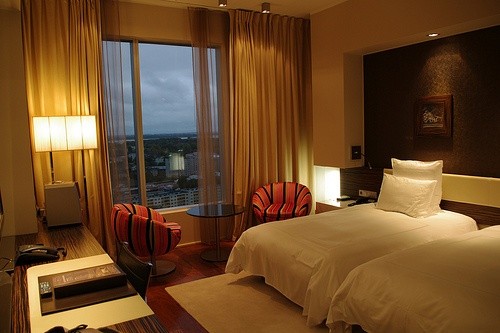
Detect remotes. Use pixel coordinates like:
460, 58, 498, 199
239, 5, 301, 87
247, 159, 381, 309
40, 281, 52, 298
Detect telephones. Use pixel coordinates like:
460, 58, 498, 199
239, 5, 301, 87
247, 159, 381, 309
15, 245, 60, 265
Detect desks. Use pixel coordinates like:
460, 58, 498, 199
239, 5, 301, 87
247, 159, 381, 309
186, 204, 246, 263
0, 223, 166, 333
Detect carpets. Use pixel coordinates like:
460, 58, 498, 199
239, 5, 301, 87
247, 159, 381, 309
164, 273, 323, 333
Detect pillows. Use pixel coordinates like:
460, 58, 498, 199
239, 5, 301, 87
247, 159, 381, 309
375, 158, 443, 217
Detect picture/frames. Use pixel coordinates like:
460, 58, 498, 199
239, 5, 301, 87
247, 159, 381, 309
413, 94, 453, 139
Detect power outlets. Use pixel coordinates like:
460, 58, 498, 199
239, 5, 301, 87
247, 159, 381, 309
352, 146, 361, 160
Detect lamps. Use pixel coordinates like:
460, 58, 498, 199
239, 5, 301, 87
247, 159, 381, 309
32, 114, 98, 184
219, 0, 227, 8
262, 2, 270, 14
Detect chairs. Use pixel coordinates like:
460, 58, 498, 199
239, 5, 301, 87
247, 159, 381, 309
251, 182, 312, 225
111, 204, 182, 276
225, 166, 500, 333
